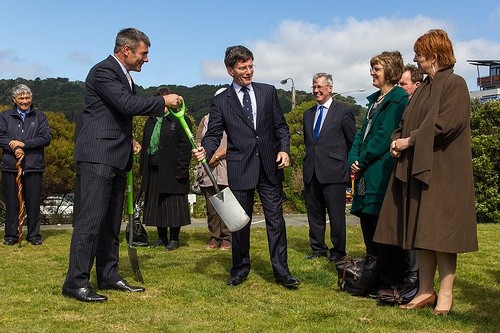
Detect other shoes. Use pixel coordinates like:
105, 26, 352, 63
218, 243, 231, 250
3, 239, 19, 245
206, 244, 218, 251
166, 239, 179, 249
153, 237, 168, 247
399, 291, 438, 310
326, 255, 346, 263
305, 253, 328, 259
30, 238, 42, 245
433, 302, 453, 315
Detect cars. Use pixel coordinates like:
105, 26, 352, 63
40, 196, 74, 219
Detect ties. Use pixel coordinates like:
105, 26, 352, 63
241, 87, 254, 128
314, 106, 325, 140
126, 73, 132, 90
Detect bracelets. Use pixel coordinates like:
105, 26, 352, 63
215, 152, 220, 161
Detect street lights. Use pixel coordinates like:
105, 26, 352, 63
280, 78, 296, 110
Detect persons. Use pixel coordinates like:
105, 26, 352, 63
348, 50, 409, 271
0, 84, 52, 245
396, 62, 423, 285
139, 87, 192, 250
62, 27, 182, 302
303, 73, 356, 261
190, 45, 301, 288
190, 87, 232, 251
373, 30, 478, 316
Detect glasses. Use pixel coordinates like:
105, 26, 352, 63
370, 67, 384, 72
310, 85, 330, 89
415, 54, 423, 59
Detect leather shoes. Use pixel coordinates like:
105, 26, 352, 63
227, 275, 247, 286
104, 279, 146, 292
62, 286, 108, 303
274, 275, 301, 287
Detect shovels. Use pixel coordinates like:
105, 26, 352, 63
167, 95, 251, 233
126, 147, 145, 284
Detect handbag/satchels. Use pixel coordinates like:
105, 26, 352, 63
335, 255, 378, 291
376, 285, 418, 305
191, 182, 205, 195
125, 191, 148, 246
